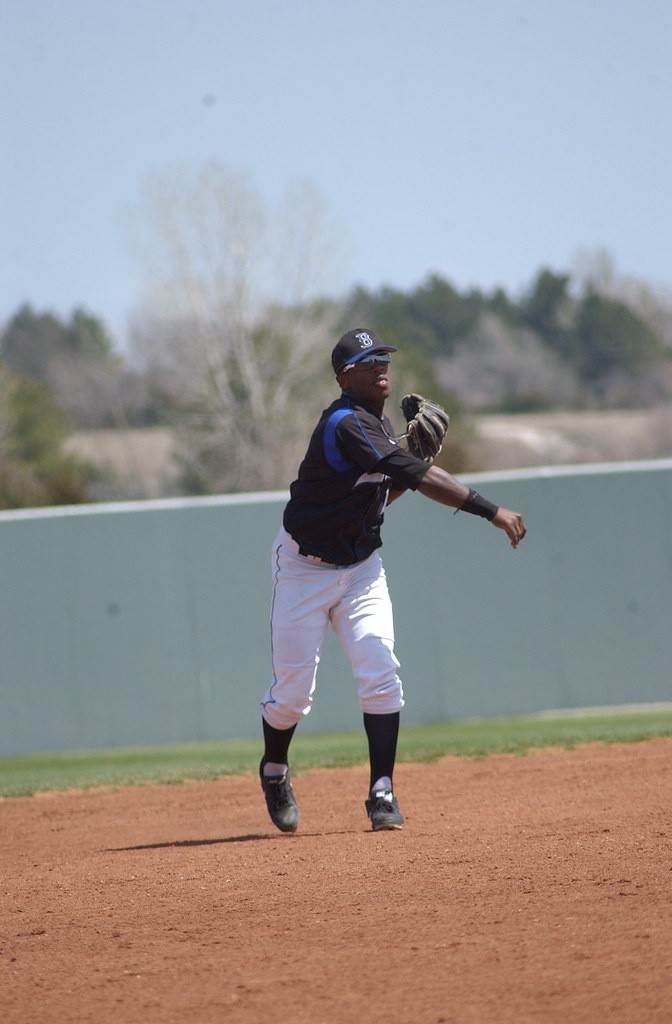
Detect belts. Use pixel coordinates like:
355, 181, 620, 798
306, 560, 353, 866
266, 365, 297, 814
298, 546, 335, 564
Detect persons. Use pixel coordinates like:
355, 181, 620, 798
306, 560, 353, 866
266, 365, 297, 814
259, 328, 527, 832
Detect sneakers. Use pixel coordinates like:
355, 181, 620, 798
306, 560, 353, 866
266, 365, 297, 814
261, 769, 300, 833
366, 790, 404, 830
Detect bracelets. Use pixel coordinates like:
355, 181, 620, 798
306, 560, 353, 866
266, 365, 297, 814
460, 487, 498, 521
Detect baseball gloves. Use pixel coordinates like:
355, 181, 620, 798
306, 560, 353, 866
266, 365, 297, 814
398, 392, 451, 464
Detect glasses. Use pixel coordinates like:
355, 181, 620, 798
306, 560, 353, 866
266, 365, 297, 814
341, 351, 391, 372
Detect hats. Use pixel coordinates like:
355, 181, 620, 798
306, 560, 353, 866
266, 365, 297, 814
332, 329, 398, 375
